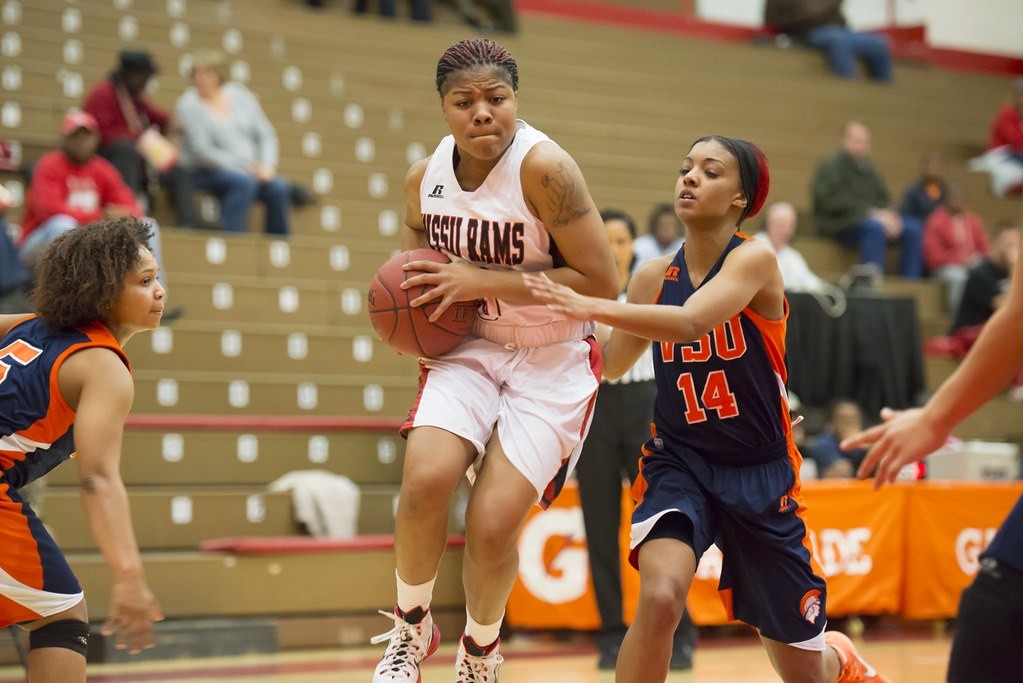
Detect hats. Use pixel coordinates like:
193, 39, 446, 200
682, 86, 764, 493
62, 110, 98, 136
116, 48, 156, 74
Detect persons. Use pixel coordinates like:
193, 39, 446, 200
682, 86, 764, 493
572, 206, 699, 671
754, 199, 826, 295
80, 51, 173, 211
838, 215, 1023, 683
0, 215, 165, 683
807, 117, 927, 286
370, 41, 624, 681
922, 199, 1023, 400
628, 204, 686, 262
987, 94, 1023, 195
526, 137, 884, 683
16, 109, 165, 288
784, 400, 961, 481
168, 50, 293, 234
763, 1, 895, 85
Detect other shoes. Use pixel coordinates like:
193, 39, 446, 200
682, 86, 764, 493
598, 641, 619, 669
670, 643, 694, 671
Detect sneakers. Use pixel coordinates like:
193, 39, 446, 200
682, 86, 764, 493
827, 632, 886, 683
371, 606, 441, 683
455, 631, 504, 683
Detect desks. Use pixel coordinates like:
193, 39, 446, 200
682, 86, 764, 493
498, 479, 1020, 646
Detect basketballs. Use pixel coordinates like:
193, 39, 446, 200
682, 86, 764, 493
367, 247, 478, 360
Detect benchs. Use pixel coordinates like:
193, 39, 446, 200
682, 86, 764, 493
0, 0, 1023, 623
